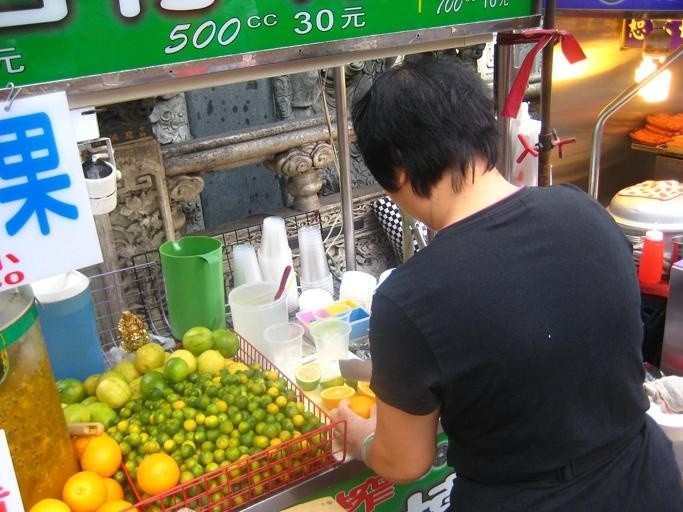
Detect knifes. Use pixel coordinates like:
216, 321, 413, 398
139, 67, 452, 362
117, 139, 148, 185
338, 356, 373, 382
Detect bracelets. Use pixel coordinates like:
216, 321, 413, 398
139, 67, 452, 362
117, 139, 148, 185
360, 430, 374, 469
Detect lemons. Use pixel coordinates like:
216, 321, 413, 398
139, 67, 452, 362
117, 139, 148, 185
28, 430, 180, 512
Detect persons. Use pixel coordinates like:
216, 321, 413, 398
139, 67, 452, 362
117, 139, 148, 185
329, 51, 681, 511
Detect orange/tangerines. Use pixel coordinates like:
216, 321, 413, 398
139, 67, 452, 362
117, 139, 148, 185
56, 326, 329, 512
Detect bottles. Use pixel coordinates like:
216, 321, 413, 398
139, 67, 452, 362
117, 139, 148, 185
81, 138, 112, 180
639, 231, 664, 284
29, 268, 107, 380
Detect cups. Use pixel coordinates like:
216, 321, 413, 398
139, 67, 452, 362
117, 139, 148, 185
297, 226, 335, 309
338, 265, 396, 312
231, 215, 299, 313
226, 280, 306, 377
159, 235, 227, 343
308, 301, 353, 377
